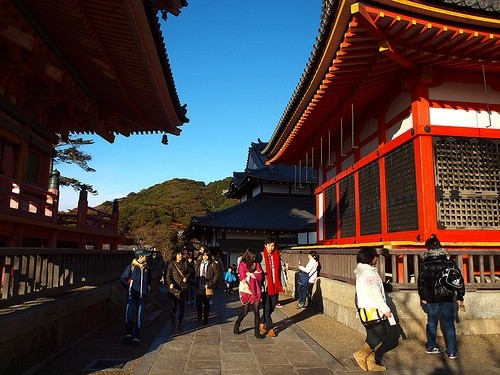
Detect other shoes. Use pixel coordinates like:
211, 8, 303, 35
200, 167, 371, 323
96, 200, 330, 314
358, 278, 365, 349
259, 323, 268, 333
203, 318, 209, 325
171, 317, 175, 325
198, 317, 202, 322
178, 323, 183, 329
269, 328, 276, 337
275, 303, 283, 308
132, 338, 141, 347
295, 302, 305, 309
122, 334, 132, 344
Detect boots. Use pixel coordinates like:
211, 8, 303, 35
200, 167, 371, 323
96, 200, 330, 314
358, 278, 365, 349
353, 342, 373, 371
254, 328, 263, 339
366, 352, 387, 372
234, 322, 242, 334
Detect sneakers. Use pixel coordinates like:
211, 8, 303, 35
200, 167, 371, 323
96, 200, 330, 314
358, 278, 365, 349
448, 352, 459, 359
426, 344, 444, 354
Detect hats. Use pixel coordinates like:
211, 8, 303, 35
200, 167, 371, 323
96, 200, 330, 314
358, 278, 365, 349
135, 249, 151, 256
425, 238, 441, 249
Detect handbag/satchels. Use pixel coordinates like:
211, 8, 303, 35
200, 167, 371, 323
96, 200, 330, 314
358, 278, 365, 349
239, 281, 252, 295
439, 257, 465, 298
206, 288, 215, 299
297, 272, 309, 287
356, 308, 383, 329
109, 267, 132, 307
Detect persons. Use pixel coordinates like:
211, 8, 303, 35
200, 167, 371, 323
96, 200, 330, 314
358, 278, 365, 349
353, 247, 391, 372
195, 246, 223, 325
275, 303, 284, 308
417, 237, 466, 359
150, 251, 166, 293
120, 249, 151, 343
234, 247, 265, 339
297, 251, 319, 308
260, 236, 287, 337
166, 248, 191, 329
224, 267, 237, 295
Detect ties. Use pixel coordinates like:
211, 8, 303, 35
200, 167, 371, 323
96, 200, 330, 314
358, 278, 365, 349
201, 263, 207, 284
